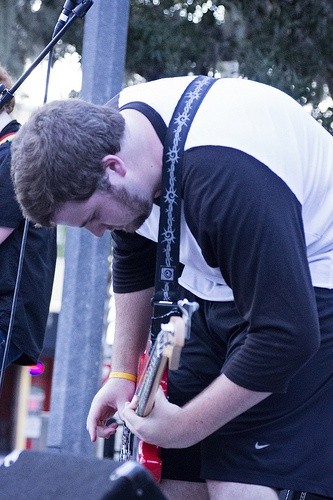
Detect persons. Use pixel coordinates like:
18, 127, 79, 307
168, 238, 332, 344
0, 66, 58, 456
11, 75, 333, 500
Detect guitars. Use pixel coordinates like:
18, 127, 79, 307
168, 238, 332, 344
112, 297, 199, 483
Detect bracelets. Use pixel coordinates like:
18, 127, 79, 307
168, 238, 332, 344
109, 372, 137, 384
